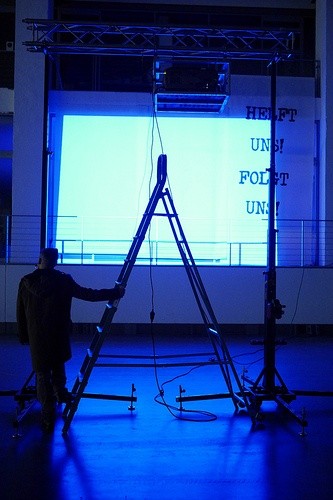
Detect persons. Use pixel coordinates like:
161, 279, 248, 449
16, 247, 126, 426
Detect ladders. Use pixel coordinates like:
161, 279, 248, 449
61, 153, 256, 436
